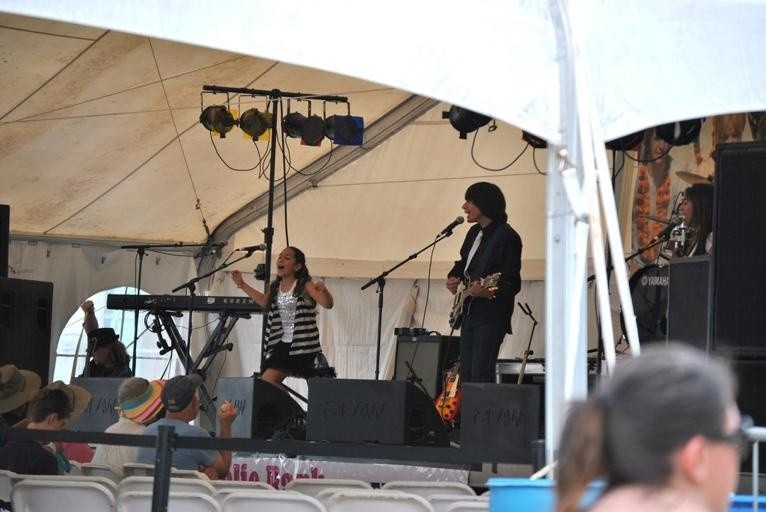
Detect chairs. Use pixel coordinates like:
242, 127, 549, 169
1, 460, 491, 511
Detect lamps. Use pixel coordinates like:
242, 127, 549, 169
199, 84, 364, 146
655, 118, 702, 147
607, 130, 645, 151
523, 130, 545, 147
443, 103, 492, 139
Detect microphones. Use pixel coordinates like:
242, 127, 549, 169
439, 217, 464, 237
237, 243, 266, 251
219, 239, 227, 248
650, 219, 678, 244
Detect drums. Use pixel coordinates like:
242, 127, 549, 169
619, 264, 669, 348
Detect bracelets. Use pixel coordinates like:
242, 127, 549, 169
237, 281, 246, 288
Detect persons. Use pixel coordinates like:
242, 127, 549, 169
556, 340, 751, 512
697, 113, 753, 178
78, 299, 134, 378
0, 365, 240, 478
446, 182, 523, 384
633, 128, 686, 263
231, 247, 334, 395
679, 183, 716, 258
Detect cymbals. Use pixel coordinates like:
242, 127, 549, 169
675, 172, 714, 185
641, 214, 673, 224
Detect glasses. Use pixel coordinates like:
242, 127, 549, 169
687, 416, 750, 461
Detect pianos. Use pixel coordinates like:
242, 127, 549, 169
107, 294, 263, 315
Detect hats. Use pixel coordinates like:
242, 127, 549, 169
465, 182, 508, 223
162, 374, 203, 412
26, 380, 91, 428
0, 364, 41, 415
85, 327, 119, 351
113, 378, 168, 425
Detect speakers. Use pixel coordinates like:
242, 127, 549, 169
0, 205, 10, 278
215, 377, 306, 442
729, 359, 766, 493
461, 382, 543, 473
392, 334, 459, 403
66, 377, 126, 433
712, 141, 765, 359
306, 376, 450, 447
1, 278, 53, 389
665, 255, 713, 364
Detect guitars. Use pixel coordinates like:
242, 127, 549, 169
449, 272, 501, 329
434, 356, 462, 421
